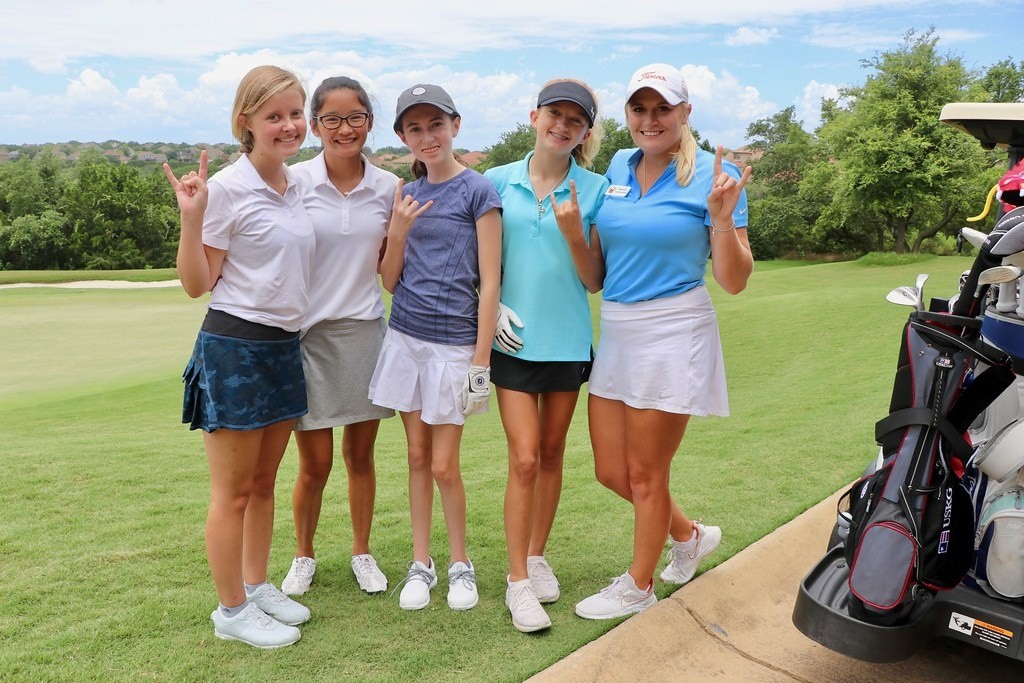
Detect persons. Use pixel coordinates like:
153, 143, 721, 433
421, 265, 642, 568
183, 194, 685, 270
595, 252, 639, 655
380, 84, 503, 611
482, 78, 610, 634
163, 65, 316, 650
573, 63, 754, 621
281, 77, 401, 596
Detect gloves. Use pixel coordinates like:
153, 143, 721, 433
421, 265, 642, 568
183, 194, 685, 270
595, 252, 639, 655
494, 301, 524, 353
462, 366, 491, 414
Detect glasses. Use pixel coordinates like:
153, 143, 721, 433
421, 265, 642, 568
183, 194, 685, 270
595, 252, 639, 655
313, 113, 370, 130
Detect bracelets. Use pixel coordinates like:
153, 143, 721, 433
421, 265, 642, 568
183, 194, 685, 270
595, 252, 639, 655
710, 214, 734, 235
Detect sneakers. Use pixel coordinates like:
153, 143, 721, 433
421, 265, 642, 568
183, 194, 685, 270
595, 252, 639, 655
447, 557, 478, 611
399, 557, 437, 610
505, 574, 551, 632
525, 555, 561, 603
244, 582, 311, 626
211, 602, 302, 650
351, 554, 388, 592
575, 571, 659, 619
660, 523, 721, 583
281, 556, 316, 595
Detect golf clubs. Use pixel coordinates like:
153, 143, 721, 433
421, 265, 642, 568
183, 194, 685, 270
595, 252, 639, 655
961, 265, 1024, 337
885, 272, 934, 313
959, 227, 989, 250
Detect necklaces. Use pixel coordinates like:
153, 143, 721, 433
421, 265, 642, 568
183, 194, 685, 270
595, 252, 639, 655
325, 159, 362, 196
642, 154, 673, 192
529, 154, 570, 219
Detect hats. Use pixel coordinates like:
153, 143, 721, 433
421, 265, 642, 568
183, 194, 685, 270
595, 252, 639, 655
625, 63, 689, 106
395, 84, 457, 123
537, 82, 596, 128
